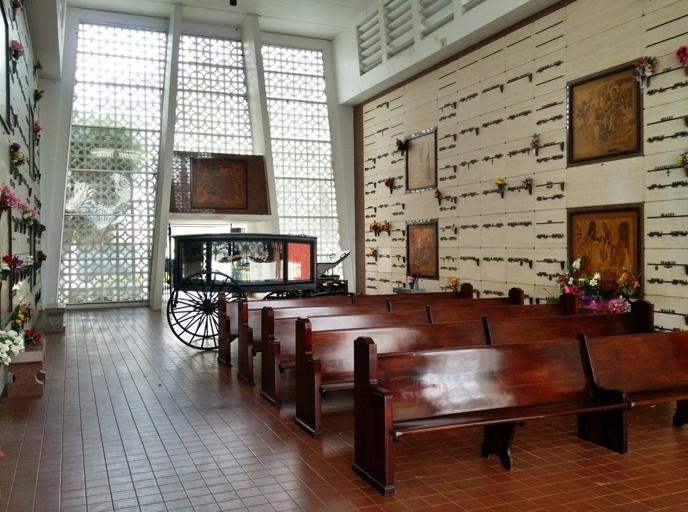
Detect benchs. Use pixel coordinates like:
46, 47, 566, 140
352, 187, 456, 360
218, 284, 687, 496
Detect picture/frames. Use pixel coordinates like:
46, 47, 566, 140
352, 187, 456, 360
189, 157, 248, 209
405, 124, 440, 197
561, 197, 651, 297
563, 57, 646, 172
404, 215, 442, 283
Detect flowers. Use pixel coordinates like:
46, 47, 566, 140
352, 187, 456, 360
444, 274, 462, 292
632, 43, 688, 178
492, 131, 545, 203
0, 0, 47, 366
551, 261, 651, 312
366, 132, 405, 262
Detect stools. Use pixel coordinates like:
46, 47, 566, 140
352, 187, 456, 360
43, 302, 67, 333
6, 350, 46, 397
